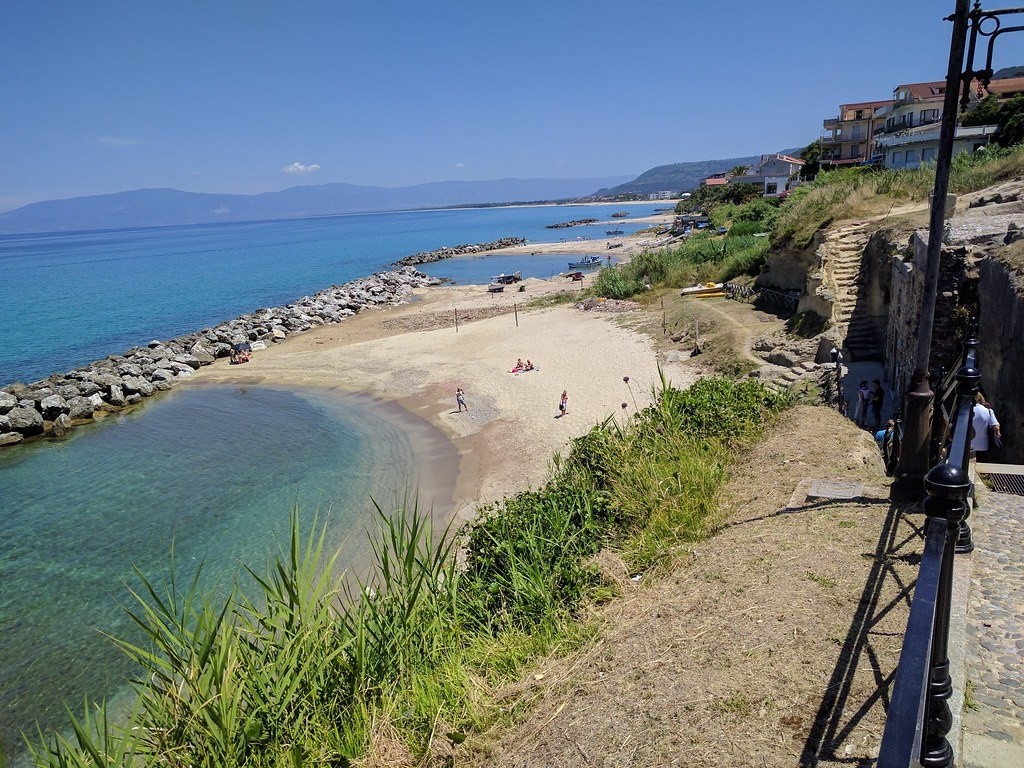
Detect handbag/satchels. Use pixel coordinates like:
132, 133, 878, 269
560, 404, 564, 410
987, 408, 1003, 450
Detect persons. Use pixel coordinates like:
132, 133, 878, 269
524, 359, 533, 370
520, 223, 684, 265
870, 379, 884, 430
561, 390, 568, 416
516, 358, 524, 370
456, 387, 468, 411
859, 421, 873, 434
969, 392, 1000, 463
854, 380, 871, 426
874, 419, 903, 462
939, 423, 956, 461
229, 348, 253, 364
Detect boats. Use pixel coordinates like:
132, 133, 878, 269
488, 282, 504, 293
491, 271, 522, 284
568, 255, 603, 268
604, 227, 624, 235
560, 269, 585, 281
